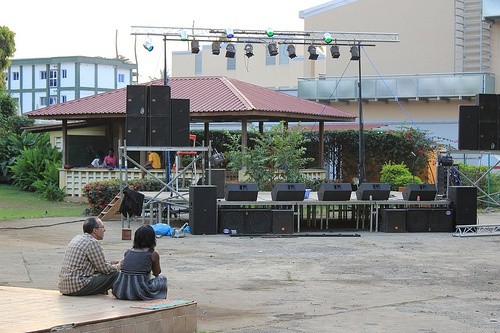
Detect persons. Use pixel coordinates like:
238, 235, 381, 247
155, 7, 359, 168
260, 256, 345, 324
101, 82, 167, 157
91, 148, 162, 180
58, 218, 119, 296
110, 224, 167, 299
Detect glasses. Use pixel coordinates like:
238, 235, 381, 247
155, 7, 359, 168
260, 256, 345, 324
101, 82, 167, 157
94, 225, 104, 228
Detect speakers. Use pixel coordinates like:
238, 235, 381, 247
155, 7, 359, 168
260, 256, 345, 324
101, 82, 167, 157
317, 183, 352, 201
147, 85, 171, 146
224, 184, 258, 201
379, 208, 406, 233
126, 85, 147, 146
171, 99, 190, 146
448, 186, 477, 232
476, 94, 500, 150
271, 183, 306, 201
189, 185, 218, 234
356, 183, 390, 200
458, 104, 479, 150
406, 209, 455, 232
218, 209, 294, 235
205, 169, 226, 198
402, 184, 437, 201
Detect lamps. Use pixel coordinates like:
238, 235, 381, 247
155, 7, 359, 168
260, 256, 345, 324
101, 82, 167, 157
330, 46, 340, 59
143, 42, 153, 51
225, 44, 236, 58
307, 46, 319, 60
266, 27, 274, 37
181, 31, 188, 40
212, 42, 221, 55
287, 45, 296, 59
323, 33, 332, 43
244, 44, 254, 58
226, 26, 234, 38
350, 46, 360, 60
191, 40, 200, 54
268, 43, 278, 57
